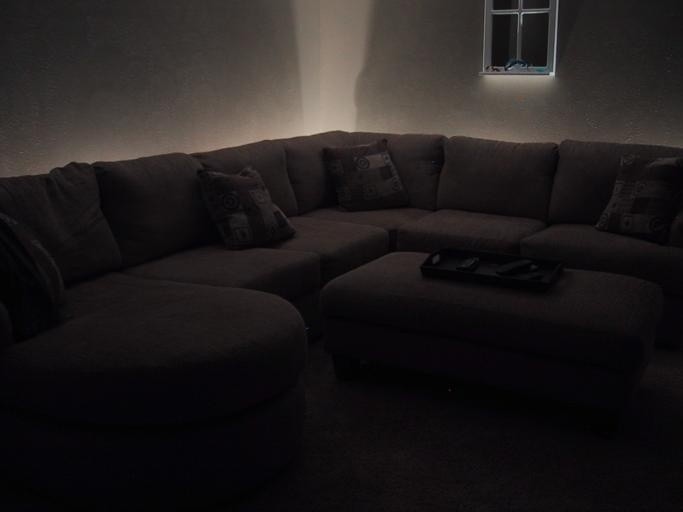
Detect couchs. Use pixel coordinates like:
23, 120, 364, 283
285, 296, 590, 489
3, 128, 683, 512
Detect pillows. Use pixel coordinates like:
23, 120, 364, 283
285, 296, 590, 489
321, 137, 410, 211
193, 165, 296, 249
597, 152, 682, 248
